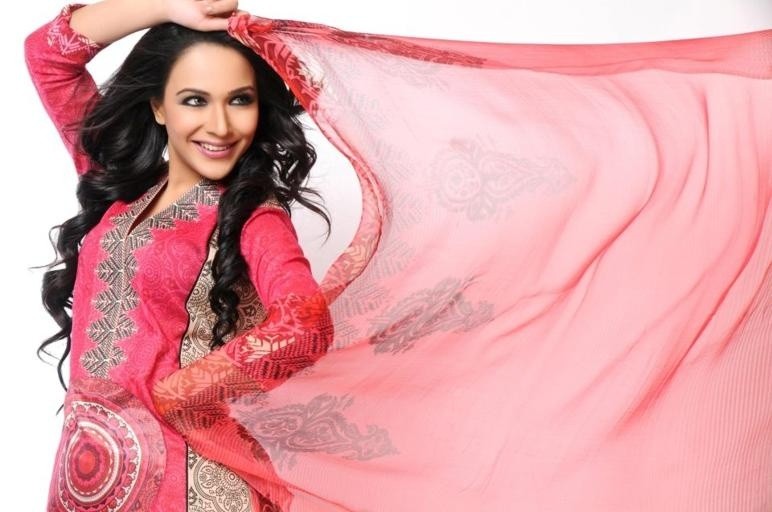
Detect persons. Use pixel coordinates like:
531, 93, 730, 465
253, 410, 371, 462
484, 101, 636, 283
24, 1, 335, 512
